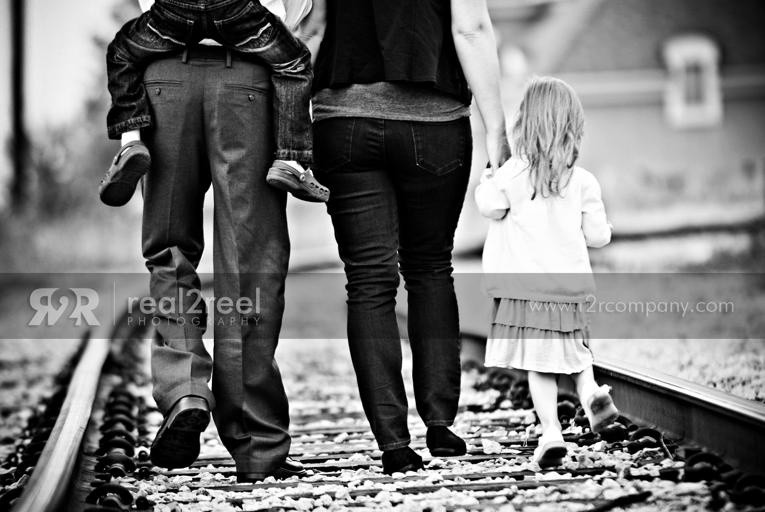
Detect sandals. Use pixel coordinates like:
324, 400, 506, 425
267, 159, 332, 203
584, 387, 619, 434
534, 435, 567, 467
98, 140, 153, 207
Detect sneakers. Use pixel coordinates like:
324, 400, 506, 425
151, 395, 209, 468
427, 426, 467, 457
237, 450, 306, 483
381, 447, 423, 474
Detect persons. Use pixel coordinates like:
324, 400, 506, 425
238, 17, 239, 21
142, 0, 317, 482
300, 0, 512, 477
474, 78, 618, 468
98, 0, 331, 207
